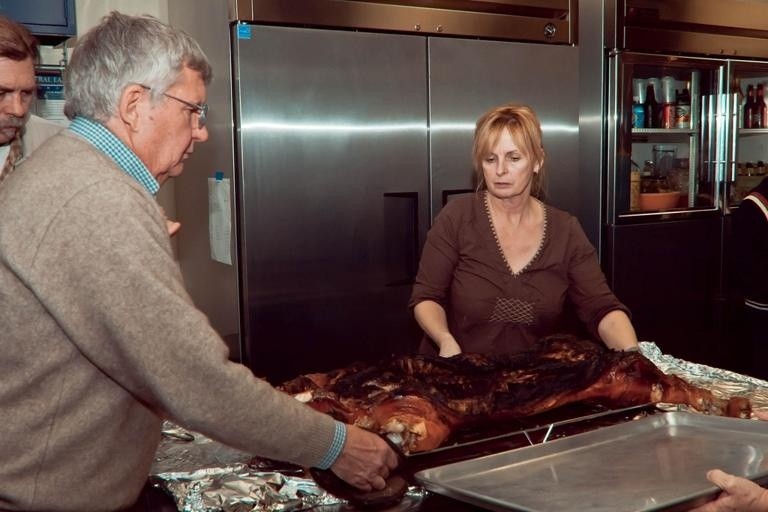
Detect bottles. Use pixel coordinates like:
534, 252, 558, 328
744, 83, 768, 129
632, 76, 692, 129
655, 143, 678, 176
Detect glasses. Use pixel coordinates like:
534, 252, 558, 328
123, 82, 209, 131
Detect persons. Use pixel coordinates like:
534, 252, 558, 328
0, 17, 402, 511
682, 468, 768, 512
1, 13, 65, 182
728, 176, 768, 383
409, 102, 646, 361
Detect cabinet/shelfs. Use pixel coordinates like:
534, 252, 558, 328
581, 1, 768, 305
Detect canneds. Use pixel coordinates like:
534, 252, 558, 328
639, 171, 654, 194
630, 76, 692, 130
644, 160, 656, 174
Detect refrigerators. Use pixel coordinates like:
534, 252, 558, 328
168, 0, 604, 388
